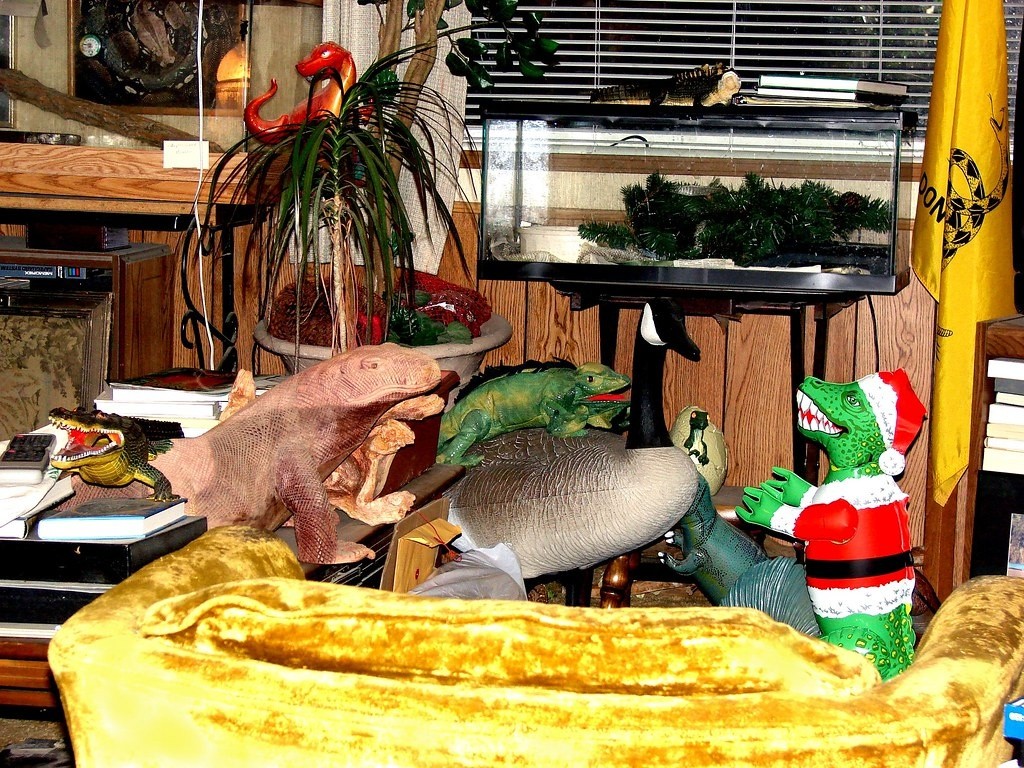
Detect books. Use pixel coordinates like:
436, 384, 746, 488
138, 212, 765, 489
982, 357, 1024, 475
94, 367, 289, 438
732, 74, 909, 108
0, 471, 208, 638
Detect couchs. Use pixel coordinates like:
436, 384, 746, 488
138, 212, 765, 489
48, 528, 1024, 768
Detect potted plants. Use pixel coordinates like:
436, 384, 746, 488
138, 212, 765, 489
169, 0, 564, 417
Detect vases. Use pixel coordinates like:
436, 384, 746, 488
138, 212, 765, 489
377, 370, 460, 498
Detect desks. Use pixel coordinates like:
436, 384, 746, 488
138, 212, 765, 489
551, 281, 870, 603
0, 191, 267, 373
0, 464, 466, 706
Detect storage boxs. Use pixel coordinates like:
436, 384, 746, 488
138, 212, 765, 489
477, 102, 919, 295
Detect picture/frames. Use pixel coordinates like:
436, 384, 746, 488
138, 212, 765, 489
66, 0, 252, 117
0, 15, 17, 129
0, 290, 114, 443
